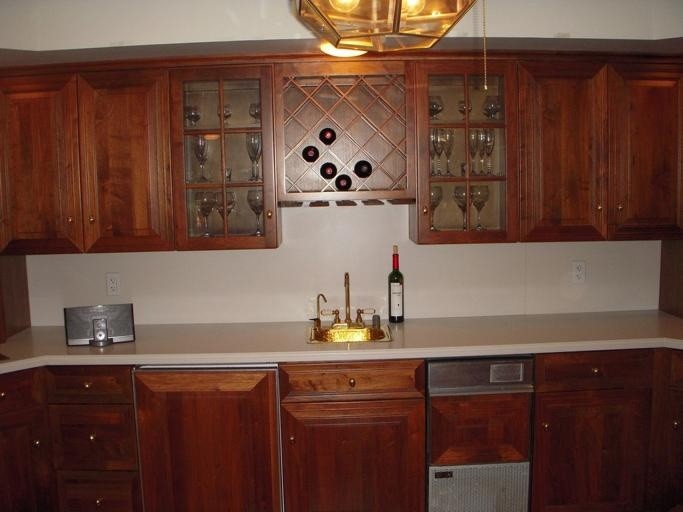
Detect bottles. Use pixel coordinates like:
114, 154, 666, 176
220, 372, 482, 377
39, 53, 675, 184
388, 246, 404, 323
303, 128, 372, 191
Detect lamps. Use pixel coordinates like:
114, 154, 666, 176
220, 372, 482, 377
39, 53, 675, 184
299, 1, 480, 53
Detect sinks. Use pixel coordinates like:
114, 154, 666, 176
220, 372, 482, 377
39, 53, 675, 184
305, 321, 393, 344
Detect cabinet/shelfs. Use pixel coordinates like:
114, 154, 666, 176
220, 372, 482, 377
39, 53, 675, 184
0, 60, 173, 252
656, 347, 683, 512
135, 366, 281, 512
170, 55, 281, 251
43, 363, 142, 511
406, 56, 516, 245
0, 368, 59, 512
516, 60, 682, 243
282, 359, 428, 512
533, 346, 655, 512
426, 359, 534, 512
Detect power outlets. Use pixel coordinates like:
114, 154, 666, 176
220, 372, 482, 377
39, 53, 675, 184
572, 260, 586, 282
106, 272, 121, 296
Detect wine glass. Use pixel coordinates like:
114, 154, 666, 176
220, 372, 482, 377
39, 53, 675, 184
458, 100, 472, 121
193, 135, 210, 183
429, 95, 444, 121
246, 133, 263, 181
470, 185, 489, 231
454, 186, 472, 231
482, 94, 501, 120
247, 190, 264, 237
429, 186, 443, 231
214, 192, 235, 220
428, 128, 456, 177
216, 104, 231, 126
194, 191, 216, 235
184, 104, 201, 127
248, 103, 263, 124
469, 128, 495, 177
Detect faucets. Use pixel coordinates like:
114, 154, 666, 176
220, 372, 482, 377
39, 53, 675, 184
316, 294, 327, 319
344, 272, 352, 323
